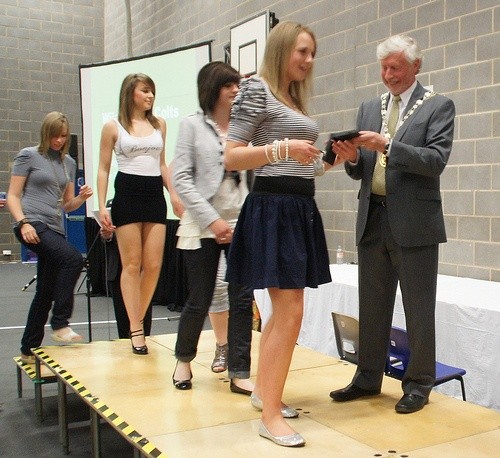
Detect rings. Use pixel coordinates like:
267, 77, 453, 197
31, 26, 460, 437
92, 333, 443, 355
221, 238, 227, 240
305, 160, 308, 164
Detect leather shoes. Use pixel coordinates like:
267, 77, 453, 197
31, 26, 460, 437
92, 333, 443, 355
330, 382, 380, 401
394, 392, 429, 414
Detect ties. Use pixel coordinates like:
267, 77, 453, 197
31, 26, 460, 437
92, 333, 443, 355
387, 95, 402, 140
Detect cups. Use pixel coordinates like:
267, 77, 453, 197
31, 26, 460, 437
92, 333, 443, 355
0, 191, 7, 206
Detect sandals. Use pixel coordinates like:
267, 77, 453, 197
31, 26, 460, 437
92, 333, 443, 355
211, 342, 229, 373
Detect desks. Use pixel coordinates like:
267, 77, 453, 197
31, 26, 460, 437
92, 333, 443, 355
253, 262, 500, 412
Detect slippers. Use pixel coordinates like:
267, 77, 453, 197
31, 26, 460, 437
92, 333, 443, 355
21, 353, 45, 364
50, 326, 84, 343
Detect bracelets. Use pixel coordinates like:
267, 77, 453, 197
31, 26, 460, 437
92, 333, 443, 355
384, 142, 391, 155
264, 137, 290, 164
17, 219, 29, 230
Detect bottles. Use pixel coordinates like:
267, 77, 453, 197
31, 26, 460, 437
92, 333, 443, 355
336, 245, 343, 264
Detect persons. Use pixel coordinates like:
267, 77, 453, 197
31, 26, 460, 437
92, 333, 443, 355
222, 22, 340, 448
6, 111, 93, 365
97, 74, 168, 355
330, 33, 456, 412
172, 61, 256, 395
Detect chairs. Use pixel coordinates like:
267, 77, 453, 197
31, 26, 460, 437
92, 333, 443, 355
331, 312, 359, 365
384, 326, 467, 402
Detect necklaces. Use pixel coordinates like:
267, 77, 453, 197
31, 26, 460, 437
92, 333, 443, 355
47, 148, 70, 218
379, 91, 438, 166
213, 118, 224, 136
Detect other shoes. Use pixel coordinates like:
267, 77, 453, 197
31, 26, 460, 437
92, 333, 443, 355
230, 378, 252, 396
172, 359, 193, 390
258, 419, 306, 447
250, 391, 299, 417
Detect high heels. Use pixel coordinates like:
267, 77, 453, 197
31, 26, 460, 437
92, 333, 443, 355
129, 319, 148, 354
